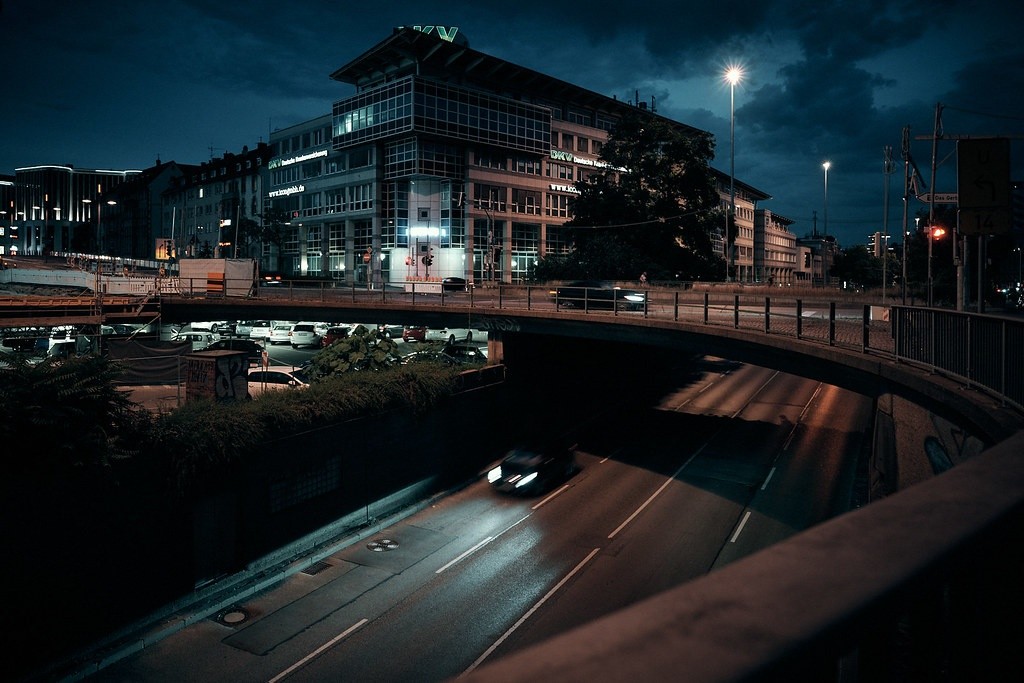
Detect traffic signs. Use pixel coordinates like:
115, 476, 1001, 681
916, 192, 958, 203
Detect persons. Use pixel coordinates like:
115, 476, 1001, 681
767, 274, 775, 286
640, 272, 647, 286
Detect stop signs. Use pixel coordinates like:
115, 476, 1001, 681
363, 254, 371, 261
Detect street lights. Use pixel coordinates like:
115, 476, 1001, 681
821, 161, 832, 290
82, 197, 116, 253
727, 68, 741, 278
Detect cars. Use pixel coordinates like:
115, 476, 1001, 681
322, 326, 348, 347
190, 319, 297, 345
441, 277, 471, 291
549, 281, 650, 310
46, 324, 148, 357
424, 327, 479, 345
403, 326, 429, 342
260, 271, 281, 286
377, 325, 404, 339
246, 365, 312, 401
288, 321, 332, 350
191, 338, 267, 358
487, 442, 577, 497
170, 331, 213, 350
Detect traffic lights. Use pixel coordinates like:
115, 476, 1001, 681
427, 259, 433, 267
868, 232, 880, 258
458, 191, 467, 208
